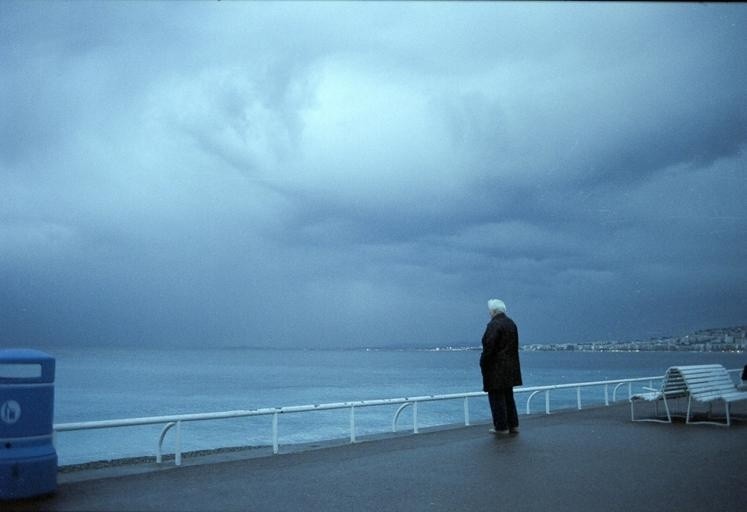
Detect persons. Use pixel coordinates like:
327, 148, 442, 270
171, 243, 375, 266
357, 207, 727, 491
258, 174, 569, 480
478, 296, 524, 436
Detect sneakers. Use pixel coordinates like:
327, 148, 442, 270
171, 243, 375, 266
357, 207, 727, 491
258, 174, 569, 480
488, 427, 520, 434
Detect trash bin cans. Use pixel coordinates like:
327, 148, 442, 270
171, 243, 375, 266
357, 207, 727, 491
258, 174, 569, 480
0, 347, 58, 500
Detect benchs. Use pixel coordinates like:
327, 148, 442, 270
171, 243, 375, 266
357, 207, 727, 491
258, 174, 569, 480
736, 364, 747, 387
676, 364, 747, 426
631, 366, 690, 423
